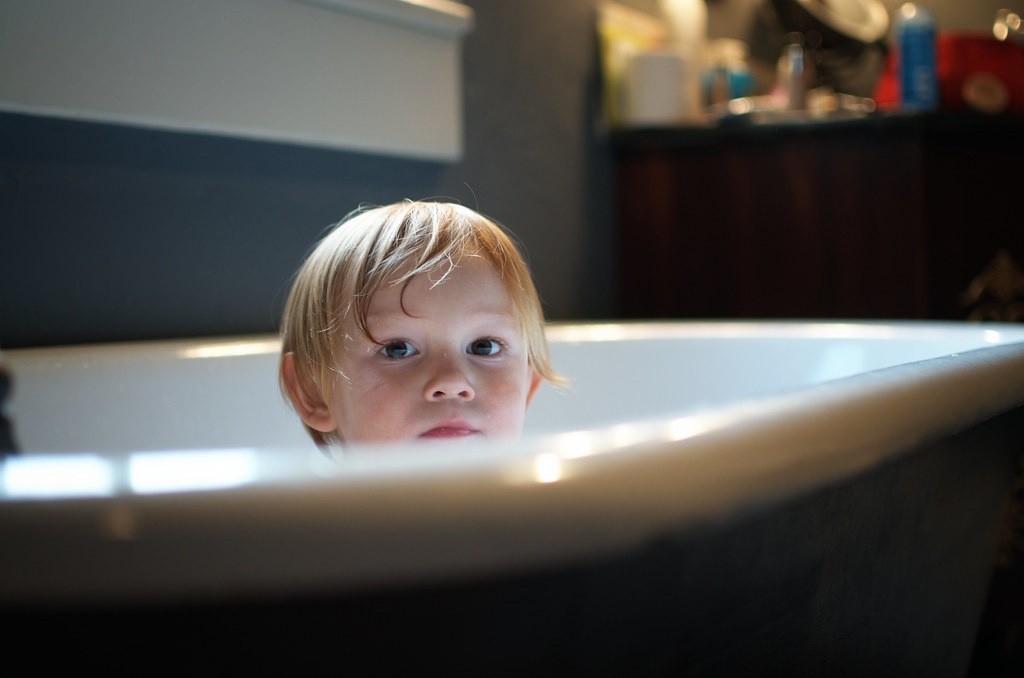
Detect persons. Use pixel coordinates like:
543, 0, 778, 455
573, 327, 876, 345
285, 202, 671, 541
277, 198, 571, 453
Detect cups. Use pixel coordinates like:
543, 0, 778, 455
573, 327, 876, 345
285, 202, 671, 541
625, 51, 705, 123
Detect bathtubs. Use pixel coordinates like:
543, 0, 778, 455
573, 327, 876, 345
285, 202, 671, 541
1, 316, 1024, 677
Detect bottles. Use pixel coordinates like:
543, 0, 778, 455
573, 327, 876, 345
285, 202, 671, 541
893, 5, 938, 115
777, 29, 809, 109
